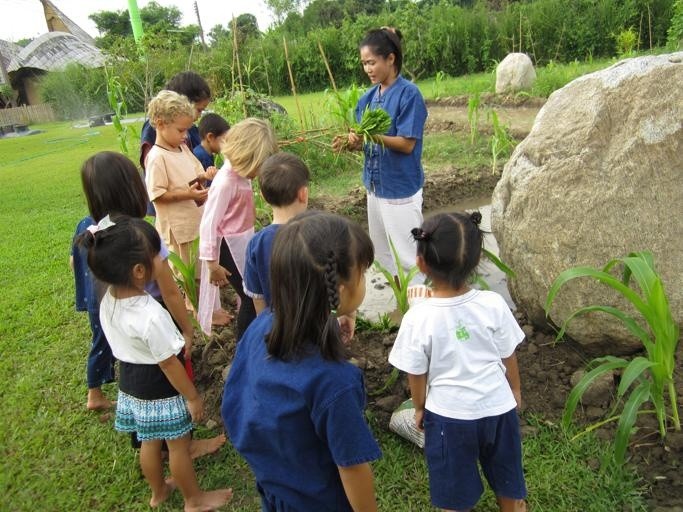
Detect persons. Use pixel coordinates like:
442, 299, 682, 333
192, 113, 230, 187
143, 90, 236, 327
138, 72, 211, 216
331, 28, 428, 292
198, 116, 278, 343
80, 150, 227, 461
77, 211, 233, 512
69, 216, 116, 410
386, 211, 527, 512
221, 209, 383, 511
240, 151, 356, 345
